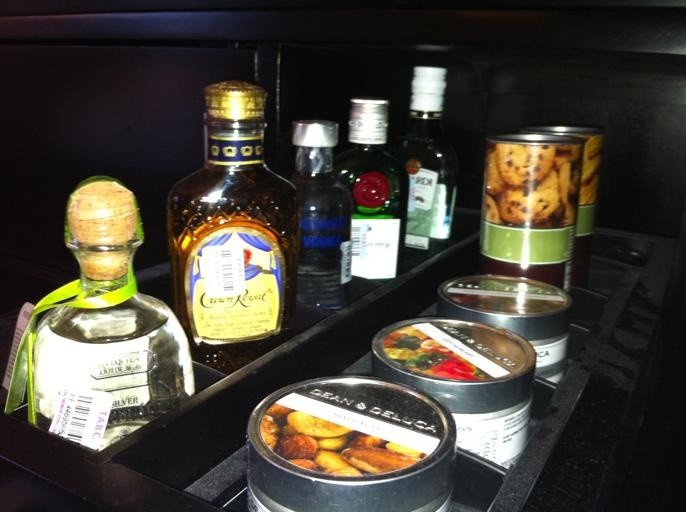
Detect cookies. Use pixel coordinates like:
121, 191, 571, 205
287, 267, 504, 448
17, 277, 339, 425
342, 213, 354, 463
484, 134, 605, 227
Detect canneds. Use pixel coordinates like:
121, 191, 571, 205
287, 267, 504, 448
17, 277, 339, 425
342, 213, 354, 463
245, 273, 573, 512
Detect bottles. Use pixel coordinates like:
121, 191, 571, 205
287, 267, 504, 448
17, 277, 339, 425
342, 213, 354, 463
26, 176, 198, 449
282, 120, 355, 314
396, 62, 466, 257
169, 82, 301, 351
340, 97, 408, 293
